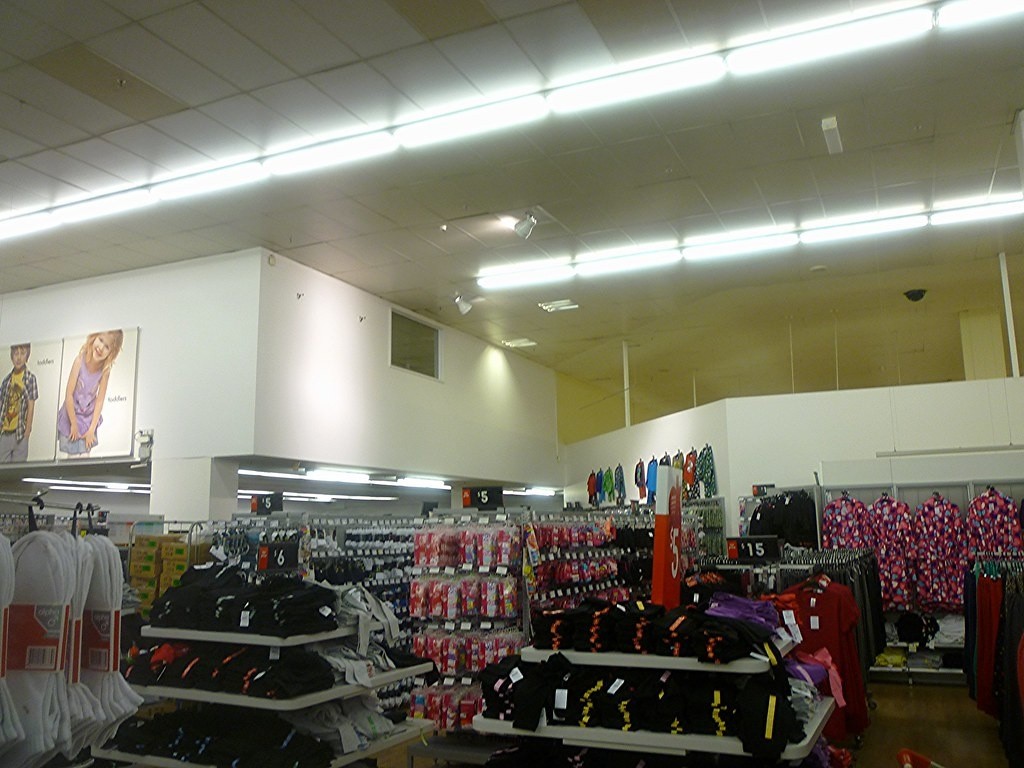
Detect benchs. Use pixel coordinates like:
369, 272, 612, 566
407, 727, 516, 768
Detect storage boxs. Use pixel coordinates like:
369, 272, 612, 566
128, 530, 215, 623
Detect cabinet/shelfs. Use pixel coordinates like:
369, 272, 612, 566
472, 633, 838, 761
89, 618, 436, 768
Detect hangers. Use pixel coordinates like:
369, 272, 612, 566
587, 442, 724, 480
586, 494, 739, 580
740, 483, 1024, 608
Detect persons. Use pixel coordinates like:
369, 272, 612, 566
58, 330, 123, 458
0, 343, 39, 463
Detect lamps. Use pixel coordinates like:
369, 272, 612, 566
514, 211, 538, 240
20, 475, 152, 496
502, 483, 557, 497
875, 443, 1024, 458
820, 116, 844, 156
451, 294, 473, 317
235, 461, 453, 504
135, 428, 152, 445
